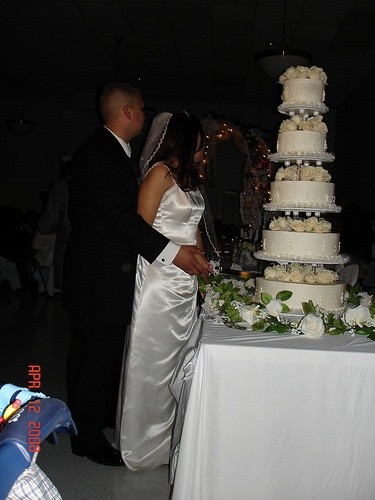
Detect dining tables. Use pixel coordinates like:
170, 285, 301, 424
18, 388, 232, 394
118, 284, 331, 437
169, 317, 374, 500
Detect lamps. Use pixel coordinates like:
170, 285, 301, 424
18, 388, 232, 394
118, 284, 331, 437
4, 80, 38, 134
253, 0, 313, 80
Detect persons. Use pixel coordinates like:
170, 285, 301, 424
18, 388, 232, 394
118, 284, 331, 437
68, 87, 209, 467
112, 111, 214, 471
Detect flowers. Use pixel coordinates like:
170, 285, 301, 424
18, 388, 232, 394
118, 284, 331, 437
197, 274, 374, 340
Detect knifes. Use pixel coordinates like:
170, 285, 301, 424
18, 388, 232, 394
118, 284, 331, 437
209, 260, 264, 279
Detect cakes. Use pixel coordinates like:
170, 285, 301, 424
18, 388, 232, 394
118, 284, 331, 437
253, 64, 349, 313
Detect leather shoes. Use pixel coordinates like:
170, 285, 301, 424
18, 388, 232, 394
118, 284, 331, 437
71, 439, 125, 466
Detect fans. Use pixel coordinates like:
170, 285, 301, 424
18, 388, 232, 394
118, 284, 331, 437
84, 37, 156, 112
